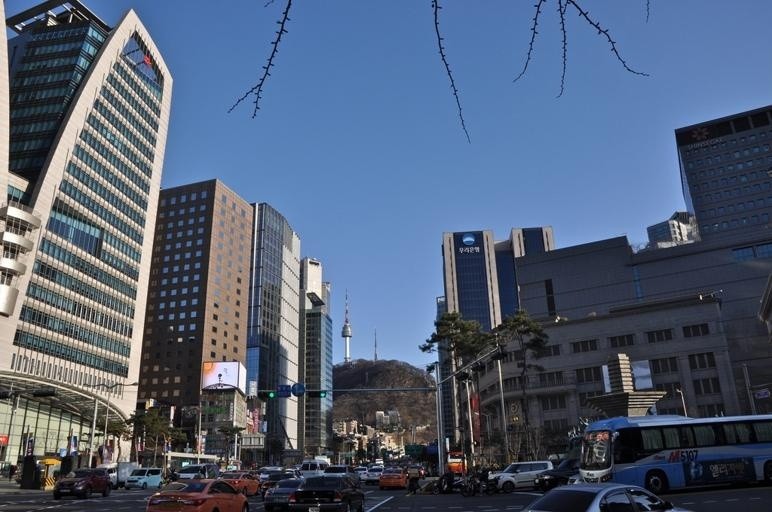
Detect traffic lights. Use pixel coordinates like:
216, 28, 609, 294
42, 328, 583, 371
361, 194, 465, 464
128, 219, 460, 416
474, 440, 480, 445
259, 392, 276, 399
308, 391, 326, 398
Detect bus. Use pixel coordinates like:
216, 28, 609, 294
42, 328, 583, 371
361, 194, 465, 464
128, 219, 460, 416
570, 413, 771, 492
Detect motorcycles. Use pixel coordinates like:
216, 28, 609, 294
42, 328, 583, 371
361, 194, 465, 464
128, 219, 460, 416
432, 474, 465, 494
460, 475, 496, 497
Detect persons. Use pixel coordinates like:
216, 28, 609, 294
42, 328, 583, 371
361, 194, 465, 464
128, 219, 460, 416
477, 466, 488, 497
406, 464, 422, 495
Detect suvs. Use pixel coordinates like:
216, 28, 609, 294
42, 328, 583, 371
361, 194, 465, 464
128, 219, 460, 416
54, 468, 111, 500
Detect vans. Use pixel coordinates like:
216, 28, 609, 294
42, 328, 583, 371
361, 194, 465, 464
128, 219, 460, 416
125, 468, 162, 490
493, 460, 553, 493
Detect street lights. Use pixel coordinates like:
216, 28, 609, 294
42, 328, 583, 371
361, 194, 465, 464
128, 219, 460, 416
675, 387, 688, 417
1, 389, 60, 476
474, 410, 501, 466
83, 382, 139, 463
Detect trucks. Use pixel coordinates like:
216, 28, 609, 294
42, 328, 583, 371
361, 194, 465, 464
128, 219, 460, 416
96, 461, 143, 489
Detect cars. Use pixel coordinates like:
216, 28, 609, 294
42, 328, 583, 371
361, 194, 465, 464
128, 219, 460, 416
146, 459, 426, 511
567, 473, 580, 485
522, 483, 693, 511
536, 456, 581, 493
